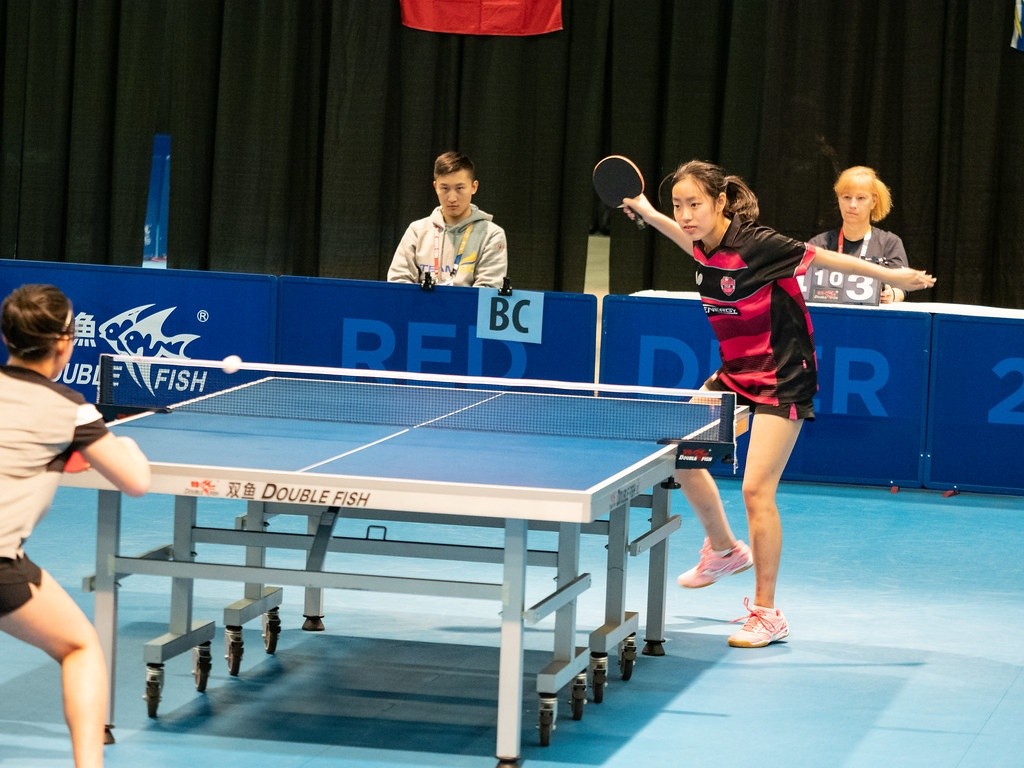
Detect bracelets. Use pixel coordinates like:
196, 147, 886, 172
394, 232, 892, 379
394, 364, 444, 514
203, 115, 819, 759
892, 289, 896, 302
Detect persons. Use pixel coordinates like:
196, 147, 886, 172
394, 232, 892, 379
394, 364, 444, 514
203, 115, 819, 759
806, 165, 909, 304
621, 161, 936, 647
0, 285, 150, 768
387, 151, 507, 288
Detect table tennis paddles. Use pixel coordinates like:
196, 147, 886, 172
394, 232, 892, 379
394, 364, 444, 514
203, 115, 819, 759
593, 155, 646, 231
64, 450, 92, 473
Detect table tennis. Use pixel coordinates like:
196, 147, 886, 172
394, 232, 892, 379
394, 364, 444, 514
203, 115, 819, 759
222, 355, 241, 372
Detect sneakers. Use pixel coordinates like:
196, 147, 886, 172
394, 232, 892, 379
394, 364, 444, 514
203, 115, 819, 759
727, 596, 789, 646
677, 537, 754, 588
